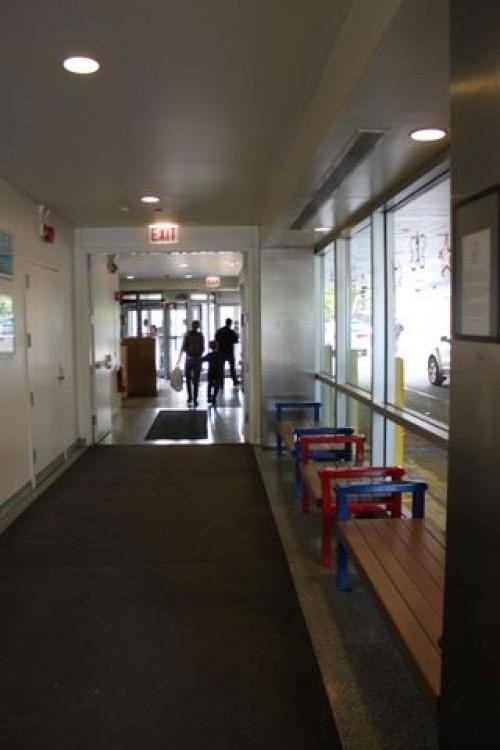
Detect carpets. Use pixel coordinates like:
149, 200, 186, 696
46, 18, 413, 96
0, 438, 344, 749
144, 411, 206, 440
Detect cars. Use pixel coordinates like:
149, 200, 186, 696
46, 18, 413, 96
426, 330, 453, 385
326, 320, 369, 357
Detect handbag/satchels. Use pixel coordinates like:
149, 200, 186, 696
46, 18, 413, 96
170, 368, 183, 391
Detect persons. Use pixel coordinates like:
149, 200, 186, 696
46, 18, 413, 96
144, 319, 157, 336
199, 340, 227, 404
216, 318, 241, 389
177, 320, 203, 408
